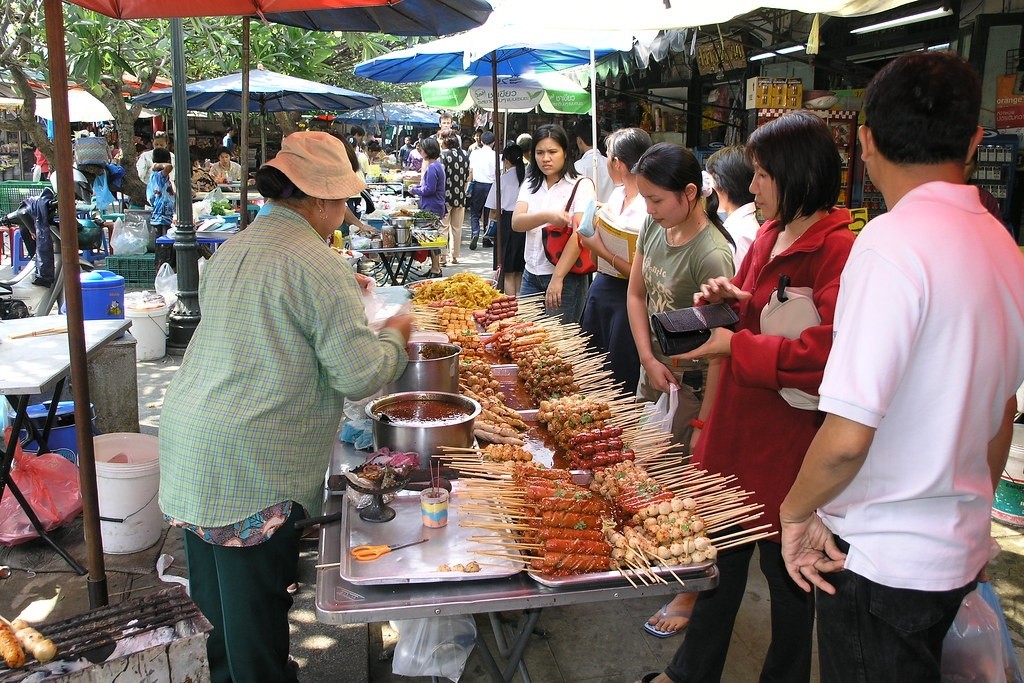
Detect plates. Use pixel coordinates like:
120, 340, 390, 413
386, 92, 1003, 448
195, 219, 240, 233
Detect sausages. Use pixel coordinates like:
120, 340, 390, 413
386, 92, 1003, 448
512, 464, 676, 575
0, 616, 25, 668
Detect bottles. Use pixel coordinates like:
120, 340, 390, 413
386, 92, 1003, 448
363, 172, 386, 183
223, 171, 232, 184
381, 225, 395, 247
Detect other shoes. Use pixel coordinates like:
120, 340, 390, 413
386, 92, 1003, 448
634, 672, 661, 683
429, 261, 447, 267
419, 269, 431, 278
427, 269, 442, 279
450, 259, 457, 264
81, 263, 93, 272
469, 234, 478, 250
483, 238, 494, 248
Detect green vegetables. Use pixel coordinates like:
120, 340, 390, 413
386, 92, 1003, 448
210, 201, 232, 216
415, 211, 436, 218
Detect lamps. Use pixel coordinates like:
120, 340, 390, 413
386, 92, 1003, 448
747, 34, 826, 63
846, 41, 929, 65
849, 0, 955, 35
707, 88, 721, 102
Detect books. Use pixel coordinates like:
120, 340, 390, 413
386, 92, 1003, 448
596, 209, 639, 280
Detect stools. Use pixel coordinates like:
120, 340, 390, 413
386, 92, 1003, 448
247, 204, 260, 224
0, 196, 125, 275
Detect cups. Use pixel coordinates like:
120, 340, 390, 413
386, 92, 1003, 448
420, 487, 449, 528
371, 241, 380, 248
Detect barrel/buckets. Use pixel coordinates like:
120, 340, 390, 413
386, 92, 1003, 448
76, 433, 163, 555
198, 216, 238, 224
124, 306, 170, 362
80, 270, 126, 338
13, 399, 103, 467
357, 260, 376, 281
394, 228, 412, 247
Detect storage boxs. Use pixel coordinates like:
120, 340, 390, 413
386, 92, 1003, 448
105, 253, 155, 289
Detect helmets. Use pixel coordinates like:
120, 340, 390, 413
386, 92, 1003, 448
77, 218, 102, 250
50, 168, 89, 194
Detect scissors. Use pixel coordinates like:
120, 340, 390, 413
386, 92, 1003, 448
352, 539, 429, 560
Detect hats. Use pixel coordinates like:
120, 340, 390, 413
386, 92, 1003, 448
259, 131, 369, 200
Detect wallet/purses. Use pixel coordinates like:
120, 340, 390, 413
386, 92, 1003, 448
652, 302, 741, 357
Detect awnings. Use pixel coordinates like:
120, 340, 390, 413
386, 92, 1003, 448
0, 87, 161, 121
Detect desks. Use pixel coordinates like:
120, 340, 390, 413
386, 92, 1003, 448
313, 411, 719, 683
156, 233, 235, 244
0, 320, 133, 575
356, 244, 447, 287
218, 183, 250, 193
193, 192, 264, 208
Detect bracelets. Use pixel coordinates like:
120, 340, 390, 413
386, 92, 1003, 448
612, 254, 617, 267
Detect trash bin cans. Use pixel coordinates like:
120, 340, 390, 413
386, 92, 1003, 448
124, 308, 168, 361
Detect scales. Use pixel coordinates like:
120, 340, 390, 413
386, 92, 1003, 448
217, 181, 241, 206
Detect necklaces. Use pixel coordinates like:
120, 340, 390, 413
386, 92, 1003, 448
671, 216, 708, 246
770, 211, 829, 260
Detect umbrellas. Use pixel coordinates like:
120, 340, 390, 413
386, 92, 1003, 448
133, 64, 382, 166
420, 72, 591, 173
42, 0, 493, 229
353, 23, 620, 270
333, 102, 456, 157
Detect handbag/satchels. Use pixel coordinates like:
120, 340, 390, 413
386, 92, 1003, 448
623, 383, 678, 465
976, 581, 1023, 683
110, 216, 149, 257
760, 276, 822, 411
196, 172, 217, 192
542, 177, 598, 275
941, 590, 1007, 683
155, 256, 208, 307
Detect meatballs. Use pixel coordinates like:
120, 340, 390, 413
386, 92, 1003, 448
441, 294, 635, 469
589, 459, 717, 571
10, 618, 57, 662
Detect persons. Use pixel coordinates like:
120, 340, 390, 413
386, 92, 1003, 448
574, 118, 623, 203
146, 147, 175, 274
348, 113, 596, 326
577, 128, 653, 414
136, 131, 175, 185
626, 143, 761, 639
34, 147, 49, 181
779, 52, 1024, 683
637, 112, 856, 683
157, 131, 413, 683
190, 126, 241, 192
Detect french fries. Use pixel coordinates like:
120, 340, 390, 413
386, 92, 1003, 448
413, 272, 505, 309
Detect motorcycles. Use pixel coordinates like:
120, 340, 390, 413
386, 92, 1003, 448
0, 170, 105, 320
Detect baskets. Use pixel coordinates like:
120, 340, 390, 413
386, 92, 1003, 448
75, 137, 112, 168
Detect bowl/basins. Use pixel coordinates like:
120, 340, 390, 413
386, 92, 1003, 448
364, 390, 482, 482
332, 248, 363, 267
404, 276, 498, 295
383, 341, 462, 397
391, 216, 440, 229
408, 333, 449, 343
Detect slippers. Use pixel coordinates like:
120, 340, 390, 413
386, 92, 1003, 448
643, 602, 693, 638
